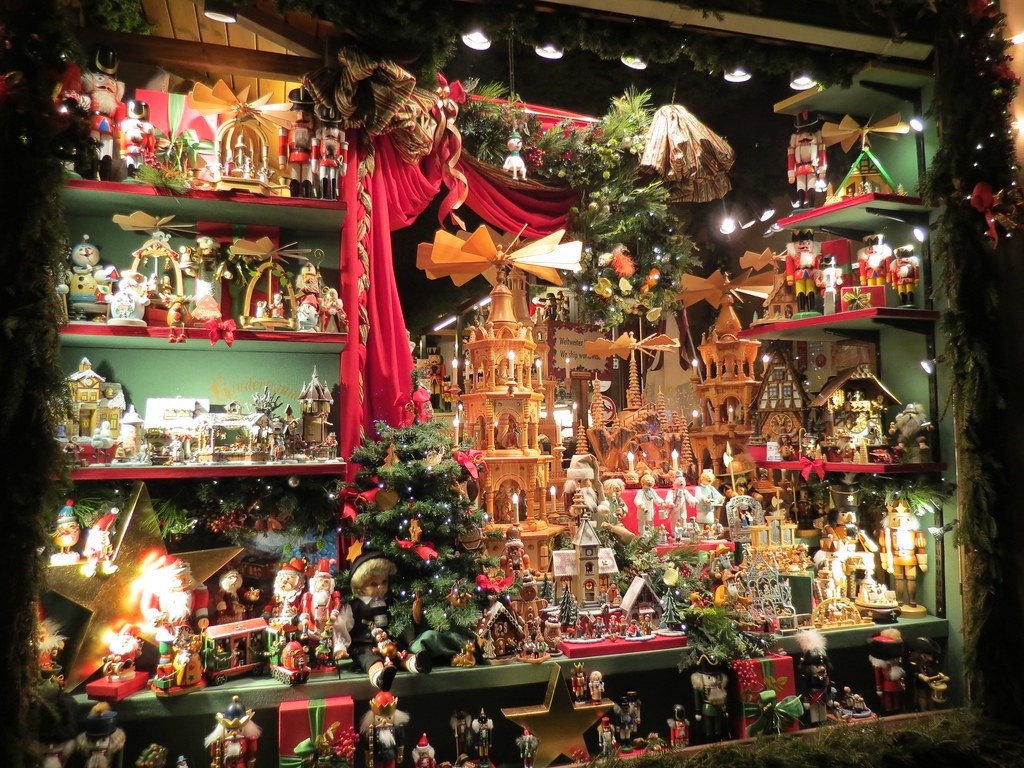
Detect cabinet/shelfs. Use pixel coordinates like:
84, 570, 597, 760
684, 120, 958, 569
51, 0, 944, 760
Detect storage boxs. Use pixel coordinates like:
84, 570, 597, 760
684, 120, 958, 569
144, 307, 170, 326
85, 672, 149, 704
278, 695, 354, 768
731, 654, 799, 739
840, 286, 886, 313
818, 238, 853, 315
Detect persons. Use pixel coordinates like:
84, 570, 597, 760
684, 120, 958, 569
62, 45, 155, 183
30, 465, 947, 768
279, 88, 349, 200
503, 131, 531, 181
420, 345, 454, 413
888, 402, 933, 456
785, 110, 919, 312
